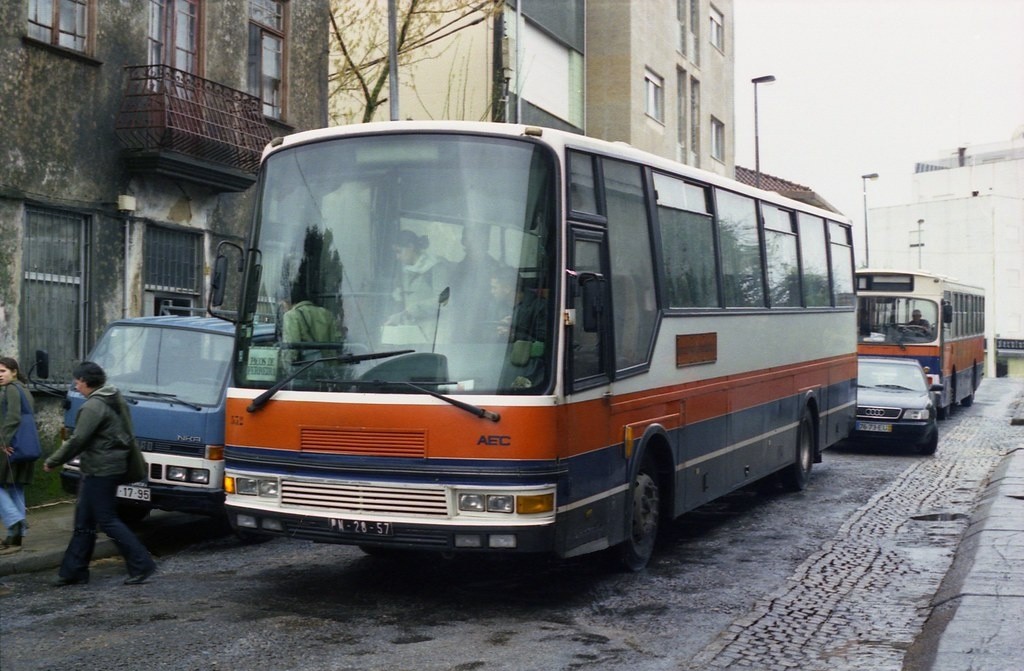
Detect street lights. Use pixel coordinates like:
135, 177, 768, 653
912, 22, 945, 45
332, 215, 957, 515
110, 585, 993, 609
862, 173, 879, 268
752, 75, 776, 188
917, 219, 925, 268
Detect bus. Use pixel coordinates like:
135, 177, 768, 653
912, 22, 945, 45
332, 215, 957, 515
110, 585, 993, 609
855, 268, 985, 420
207, 120, 857, 572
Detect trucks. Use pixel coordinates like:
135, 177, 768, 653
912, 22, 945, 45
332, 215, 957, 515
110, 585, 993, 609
28, 314, 277, 542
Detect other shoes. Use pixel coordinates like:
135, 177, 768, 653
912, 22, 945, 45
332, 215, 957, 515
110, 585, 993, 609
51, 575, 89, 586
124, 569, 155, 584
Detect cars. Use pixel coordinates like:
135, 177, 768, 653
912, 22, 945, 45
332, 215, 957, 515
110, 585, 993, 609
836, 356, 945, 454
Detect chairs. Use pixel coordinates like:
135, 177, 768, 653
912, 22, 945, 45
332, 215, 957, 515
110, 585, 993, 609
571, 273, 639, 380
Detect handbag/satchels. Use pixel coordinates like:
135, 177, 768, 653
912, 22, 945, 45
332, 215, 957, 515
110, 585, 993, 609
2, 383, 42, 462
118, 393, 148, 483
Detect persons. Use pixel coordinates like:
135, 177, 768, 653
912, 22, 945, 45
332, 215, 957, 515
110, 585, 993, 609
275, 286, 343, 391
41, 361, 158, 588
450, 223, 547, 343
0, 357, 35, 556
386, 229, 453, 327
906, 310, 930, 334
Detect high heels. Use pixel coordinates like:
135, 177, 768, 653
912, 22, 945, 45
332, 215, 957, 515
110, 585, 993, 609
2, 535, 22, 546
8, 519, 30, 545
